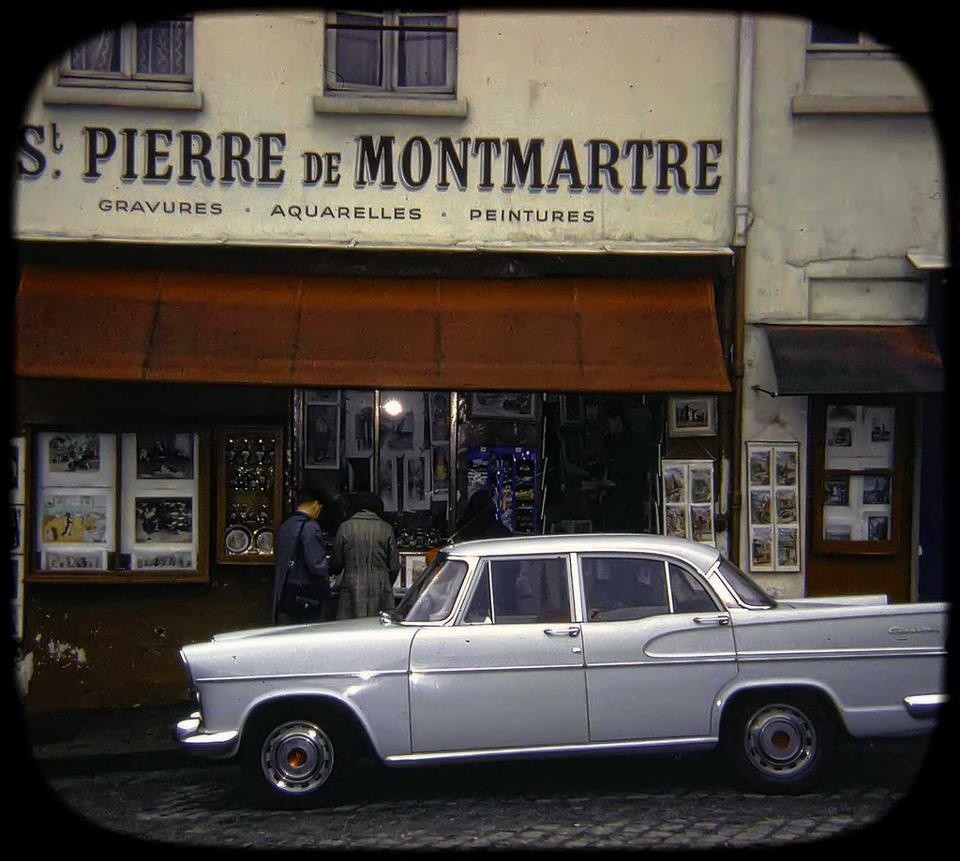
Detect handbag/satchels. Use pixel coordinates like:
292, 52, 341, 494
275, 564, 323, 625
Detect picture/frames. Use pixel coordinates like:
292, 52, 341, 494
300, 390, 544, 515
561, 393, 585, 426
666, 398, 724, 438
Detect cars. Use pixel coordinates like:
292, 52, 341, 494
173, 530, 947, 813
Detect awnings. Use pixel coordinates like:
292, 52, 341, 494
764, 323, 945, 395
11, 251, 734, 392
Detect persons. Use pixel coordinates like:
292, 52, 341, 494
459, 491, 513, 539
273, 486, 333, 626
328, 492, 401, 619
587, 404, 647, 534
